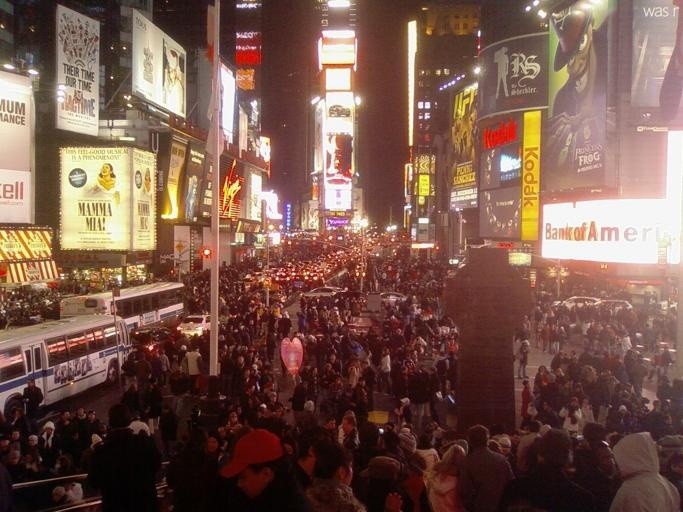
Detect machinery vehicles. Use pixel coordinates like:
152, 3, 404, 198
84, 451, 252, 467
656, 296, 677, 318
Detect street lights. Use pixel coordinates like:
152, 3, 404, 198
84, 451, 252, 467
352, 217, 370, 294
266, 232, 280, 310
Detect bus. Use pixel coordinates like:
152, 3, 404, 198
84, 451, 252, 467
0, 313, 131, 432
60, 282, 186, 334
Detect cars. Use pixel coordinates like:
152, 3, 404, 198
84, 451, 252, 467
380, 292, 408, 305
177, 314, 221, 338
242, 250, 352, 288
300, 287, 345, 298
333, 292, 369, 305
347, 313, 383, 339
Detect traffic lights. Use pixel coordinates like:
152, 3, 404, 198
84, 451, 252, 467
202, 248, 212, 257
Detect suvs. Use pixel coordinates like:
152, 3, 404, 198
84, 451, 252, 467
130, 326, 178, 358
552, 295, 601, 313
588, 300, 634, 317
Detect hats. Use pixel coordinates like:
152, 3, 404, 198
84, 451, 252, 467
219, 429, 284, 477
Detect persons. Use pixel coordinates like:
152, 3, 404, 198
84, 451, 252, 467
493, 47, 510, 98
98, 163, 116, 190
549, 0, 619, 185
1, 212, 683, 512
